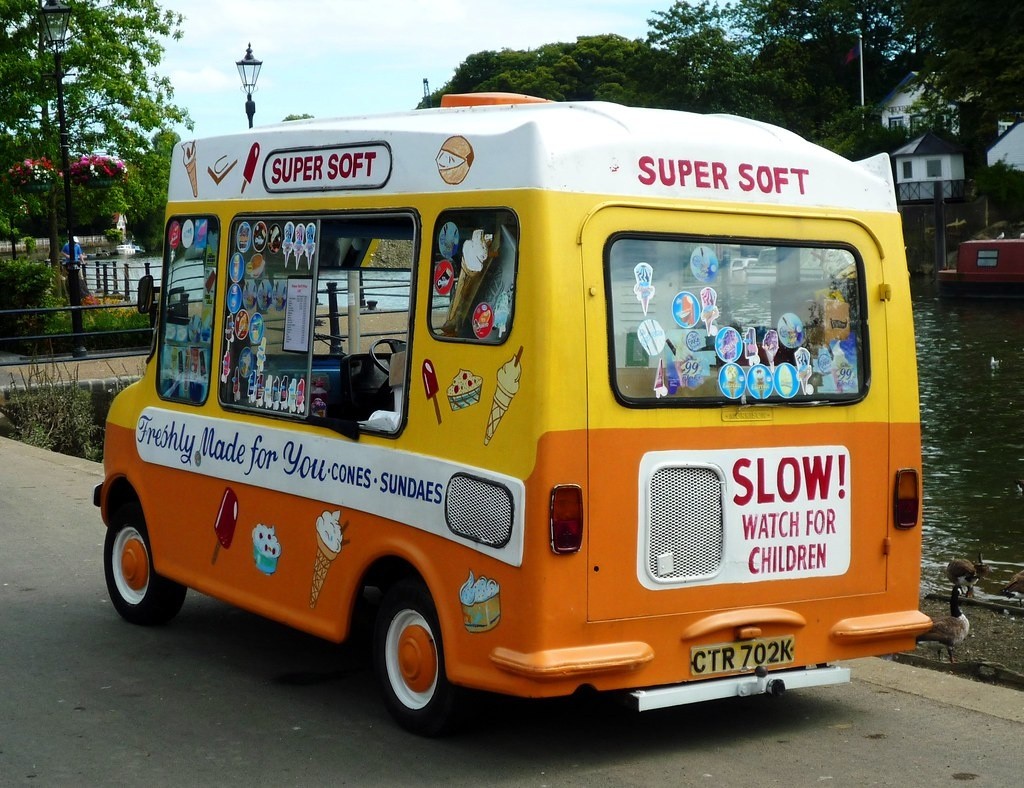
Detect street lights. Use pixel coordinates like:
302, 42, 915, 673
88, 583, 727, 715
38, 0, 90, 359
236, 43, 264, 130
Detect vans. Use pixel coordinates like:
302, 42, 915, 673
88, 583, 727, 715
91, 90, 935, 741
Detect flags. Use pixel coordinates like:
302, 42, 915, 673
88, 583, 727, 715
843, 40, 860, 65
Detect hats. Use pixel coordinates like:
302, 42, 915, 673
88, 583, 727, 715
73, 236, 79, 243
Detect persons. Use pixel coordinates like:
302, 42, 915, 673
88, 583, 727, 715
61, 236, 88, 271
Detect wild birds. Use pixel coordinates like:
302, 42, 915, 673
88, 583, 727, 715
918, 552, 1024, 663
